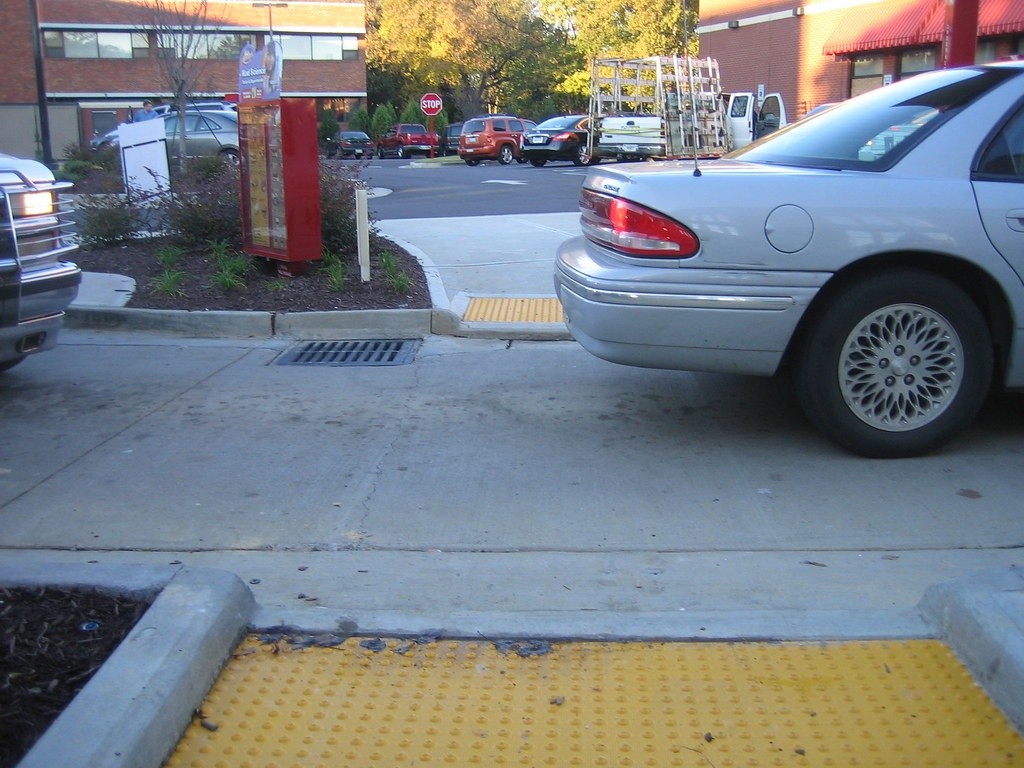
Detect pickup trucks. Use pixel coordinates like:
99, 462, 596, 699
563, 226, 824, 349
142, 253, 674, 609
598, 91, 786, 163
377, 124, 440, 159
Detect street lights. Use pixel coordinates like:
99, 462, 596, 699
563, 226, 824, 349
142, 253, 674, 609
251, 3, 289, 42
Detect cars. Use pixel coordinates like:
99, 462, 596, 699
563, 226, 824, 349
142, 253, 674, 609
552, 61, 1024, 461
94, 110, 239, 167
519, 116, 604, 166
798, 102, 929, 162
327, 132, 374, 160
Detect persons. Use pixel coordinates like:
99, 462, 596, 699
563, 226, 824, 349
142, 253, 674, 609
134, 101, 157, 122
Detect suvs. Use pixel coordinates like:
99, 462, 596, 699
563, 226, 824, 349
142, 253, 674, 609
0, 153, 81, 372
456, 114, 537, 166
89, 100, 238, 153
439, 122, 465, 157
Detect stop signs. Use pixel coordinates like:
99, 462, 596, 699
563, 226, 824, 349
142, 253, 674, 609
419, 93, 443, 116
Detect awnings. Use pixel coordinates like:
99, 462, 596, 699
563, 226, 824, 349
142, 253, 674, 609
822, 0, 1024, 56
